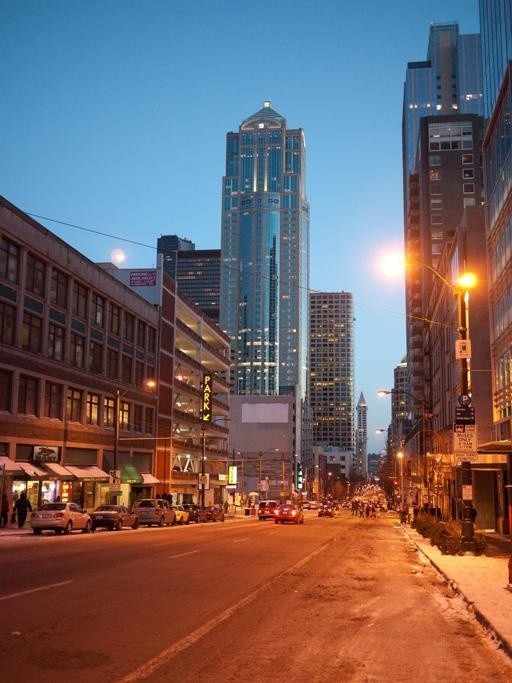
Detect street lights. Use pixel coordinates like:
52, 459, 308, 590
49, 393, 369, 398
377, 390, 427, 514
200, 416, 232, 508
380, 447, 400, 514
111, 378, 157, 505
379, 256, 482, 558
281, 450, 332, 504
258, 446, 280, 501
376, 429, 406, 511
231, 447, 241, 519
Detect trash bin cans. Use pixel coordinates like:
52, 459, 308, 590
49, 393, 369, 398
245, 509, 250, 515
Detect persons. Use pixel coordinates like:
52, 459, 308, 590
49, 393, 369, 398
2, 494, 10, 526
350, 498, 377, 518
470, 504, 476, 521
13, 493, 33, 528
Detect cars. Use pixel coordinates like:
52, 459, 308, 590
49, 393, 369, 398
26, 497, 226, 535
274, 504, 305, 523
300, 499, 388, 518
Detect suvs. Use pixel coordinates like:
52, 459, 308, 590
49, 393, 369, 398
257, 501, 280, 521
